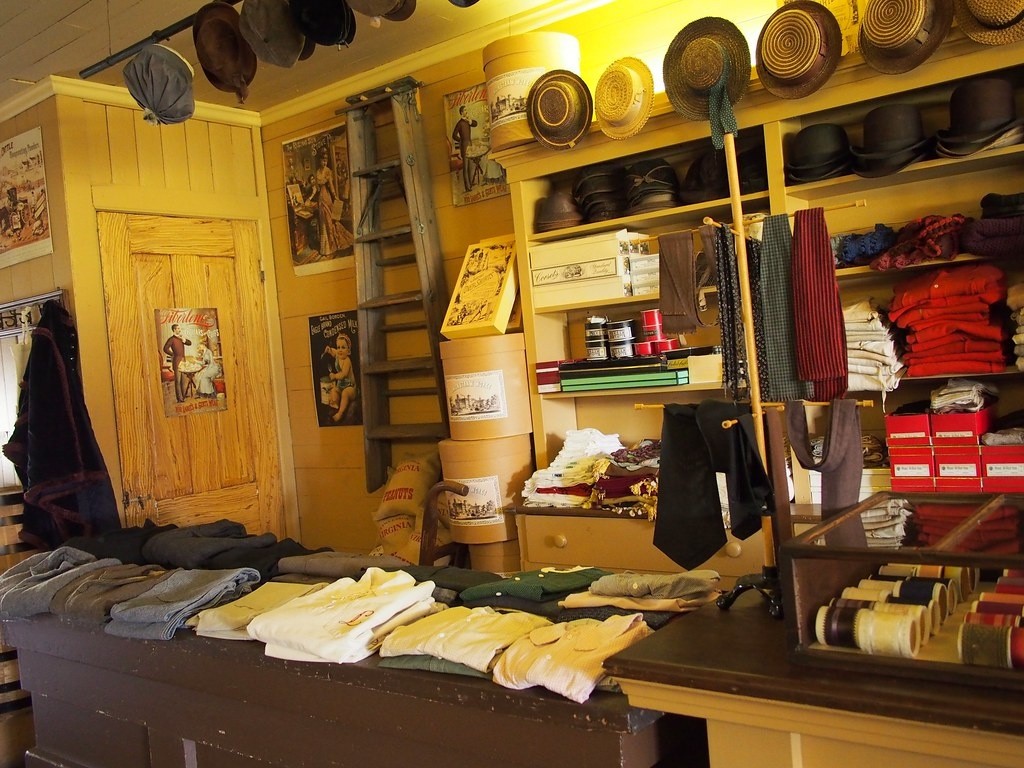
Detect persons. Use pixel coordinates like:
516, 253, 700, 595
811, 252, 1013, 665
326, 334, 357, 421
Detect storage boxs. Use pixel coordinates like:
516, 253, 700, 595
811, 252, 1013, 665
983, 476, 1024, 492
441, 239, 520, 339
888, 444, 934, 478
663, 347, 721, 369
559, 354, 669, 378
883, 402, 929, 445
527, 227, 629, 272
935, 477, 982, 492
562, 369, 688, 391
890, 478, 934, 492
530, 256, 631, 286
934, 444, 982, 478
628, 231, 639, 254
692, 355, 722, 383
931, 388, 1000, 446
532, 275, 631, 304
536, 353, 584, 395
639, 234, 648, 255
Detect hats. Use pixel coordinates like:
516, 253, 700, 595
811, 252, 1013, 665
663, 17, 751, 149
857, 0, 954, 75
954, 0, 1024, 45
595, 56, 654, 141
123, 1, 479, 132
754, 1, 843, 101
526, 69, 593, 151
519, 79, 1024, 232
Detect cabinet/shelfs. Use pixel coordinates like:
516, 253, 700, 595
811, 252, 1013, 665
982, 442, 1023, 477
617, 589, 1023, 767
486, 24, 1024, 589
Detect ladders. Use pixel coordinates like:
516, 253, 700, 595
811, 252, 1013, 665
334, 75, 452, 494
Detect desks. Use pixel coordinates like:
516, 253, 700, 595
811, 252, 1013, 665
2, 617, 666, 767
